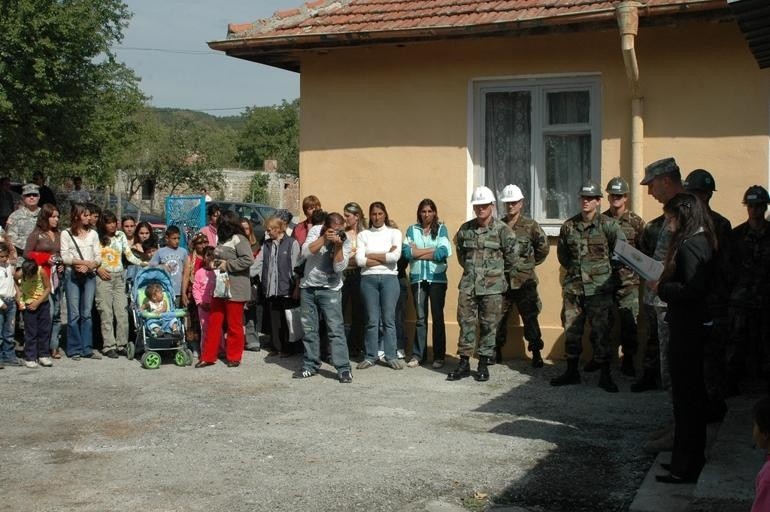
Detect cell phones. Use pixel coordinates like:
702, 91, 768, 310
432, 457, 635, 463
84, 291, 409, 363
214, 260, 222, 268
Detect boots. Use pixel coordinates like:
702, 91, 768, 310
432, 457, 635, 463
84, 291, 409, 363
584, 352, 659, 394
532, 350, 544, 367
551, 360, 580, 387
447, 356, 470, 379
477, 349, 502, 381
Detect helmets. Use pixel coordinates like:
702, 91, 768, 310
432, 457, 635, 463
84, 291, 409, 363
469, 186, 496, 206
683, 169, 715, 192
579, 179, 602, 198
497, 185, 525, 203
606, 178, 630, 196
743, 186, 770, 204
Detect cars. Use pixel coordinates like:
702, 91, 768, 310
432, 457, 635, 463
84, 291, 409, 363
171, 200, 298, 238
79, 190, 164, 228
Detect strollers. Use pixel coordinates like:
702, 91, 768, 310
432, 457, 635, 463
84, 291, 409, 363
124, 266, 195, 370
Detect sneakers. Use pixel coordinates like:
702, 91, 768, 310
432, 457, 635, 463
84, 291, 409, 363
172, 330, 181, 338
292, 367, 314, 378
157, 330, 163, 338
338, 371, 353, 383
406, 357, 421, 368
250, 344, 293, 358
0, 350, 61, 369
72, 346, 128, 361
645, 424, 676, 451
195, 353, 239, 368
432, 359, 444, 369
355, 348, 407, 370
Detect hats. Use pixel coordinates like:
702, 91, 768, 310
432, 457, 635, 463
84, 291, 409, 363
192, 234, 209, 244
639, 157, 679, 186
22, 183, 40, 195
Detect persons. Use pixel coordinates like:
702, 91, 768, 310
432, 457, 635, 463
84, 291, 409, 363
731, 184, 769, 390
640, 154, 684, 453
550, 181, 627, 392
647, 194, 730, 482
584, 177, 645, 376
495, 184, 550, 368
683, 169, 730, 245
749, 399, 770, 512
0, 169, 454, 381
446, 185, 520, 381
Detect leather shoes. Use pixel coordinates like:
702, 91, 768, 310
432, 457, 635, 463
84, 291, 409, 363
656, 462, 690, 484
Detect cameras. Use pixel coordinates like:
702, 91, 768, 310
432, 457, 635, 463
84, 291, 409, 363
51, 256, 62, 264
333, 229, 347, 242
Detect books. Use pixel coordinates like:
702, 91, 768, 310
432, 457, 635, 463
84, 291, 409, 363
613, 237, 663, 281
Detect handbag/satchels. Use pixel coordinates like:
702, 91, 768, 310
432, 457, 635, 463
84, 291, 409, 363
70, 267, 88, 282
284, 307, 305, 343
